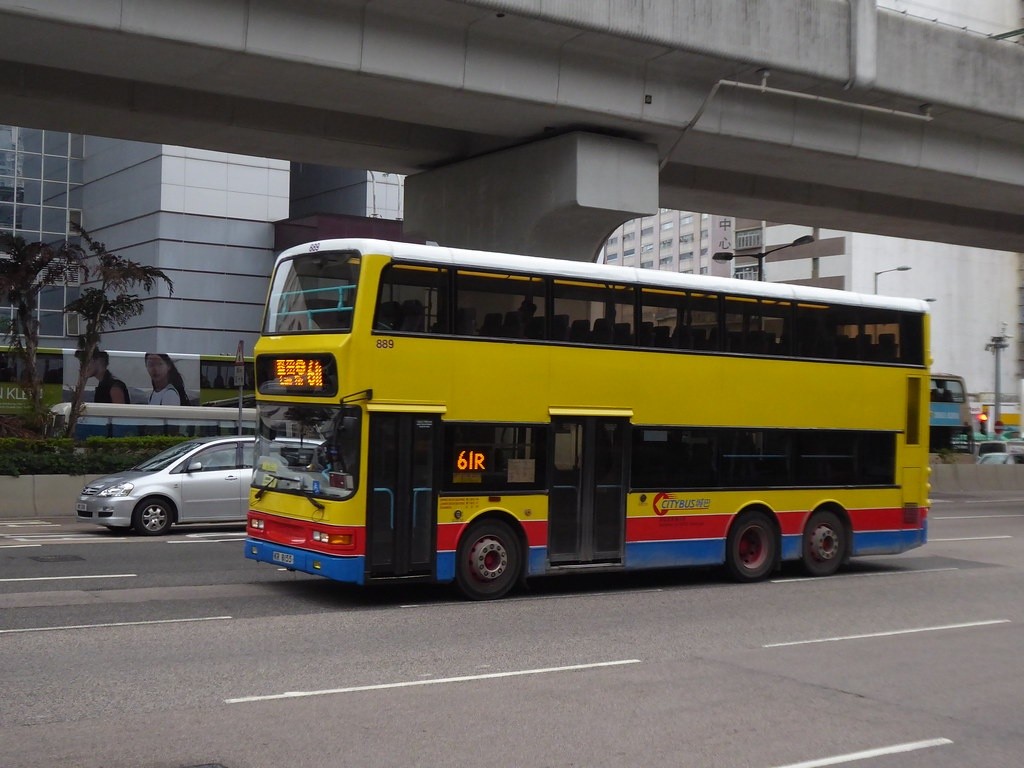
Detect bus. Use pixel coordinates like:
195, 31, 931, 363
242, 238, 934, 601
46, 402, 256, 438
0, 343, 256, 419
929, 373, 970, 453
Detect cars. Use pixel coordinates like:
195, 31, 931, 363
978, 442, 1006, 457
1006, 438, 1024, 457
973, 452, 1024, 467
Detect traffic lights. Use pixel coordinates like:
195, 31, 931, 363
975, 411, 990, 436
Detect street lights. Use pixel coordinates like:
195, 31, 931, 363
873, 265, 912, 295
985, 336, 1010, 421
711, 236, 815, 282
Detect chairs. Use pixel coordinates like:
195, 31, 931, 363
931, 387, 953, 403
202, 375, 250, 389
0, 366, 63, 386
373, 297, 901, 363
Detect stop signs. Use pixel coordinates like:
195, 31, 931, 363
994, 420, 1005, 436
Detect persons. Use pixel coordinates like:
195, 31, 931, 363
302, 423, 355, 471
74, 350, 131, 404
145, 352, 190, 405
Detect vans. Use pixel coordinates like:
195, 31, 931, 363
74, 436, 350, 537
